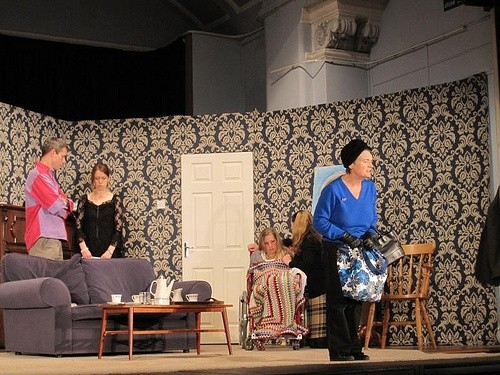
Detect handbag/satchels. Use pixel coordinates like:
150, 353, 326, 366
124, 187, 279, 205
337, 240, 390, 302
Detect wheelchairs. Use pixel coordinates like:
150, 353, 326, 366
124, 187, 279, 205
237, 249, 310, 351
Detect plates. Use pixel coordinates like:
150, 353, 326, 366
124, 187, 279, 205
107, 302, 125, 305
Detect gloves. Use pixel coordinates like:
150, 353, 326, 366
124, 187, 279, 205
365, 235, 376, 249
340, 231, 361, 248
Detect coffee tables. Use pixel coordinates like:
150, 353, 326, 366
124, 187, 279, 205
94, 301, 233, 361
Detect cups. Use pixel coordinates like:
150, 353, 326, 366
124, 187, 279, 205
139, 291, 151, 305
110, 294, 122, 303
185, 293, 199, 302
132, 295, 143, 303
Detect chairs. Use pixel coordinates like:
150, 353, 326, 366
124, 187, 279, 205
363, 243, 438, 352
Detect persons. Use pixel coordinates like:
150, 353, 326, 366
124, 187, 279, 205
25, 138, 74, 260
247, 210, 327, 349
75, 163, 122, 260
313, 139, 378, 361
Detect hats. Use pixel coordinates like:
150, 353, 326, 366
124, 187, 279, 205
340, 139, 367, 167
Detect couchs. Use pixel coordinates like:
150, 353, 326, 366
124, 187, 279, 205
0, 253, 212, 358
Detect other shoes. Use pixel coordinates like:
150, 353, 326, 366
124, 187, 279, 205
329, 352, 354, 361
353, 353, 370, 361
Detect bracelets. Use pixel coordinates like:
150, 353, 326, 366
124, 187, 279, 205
81, 248, 88, 253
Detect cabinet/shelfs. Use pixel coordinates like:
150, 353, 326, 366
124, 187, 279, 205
0, 203, 77, 258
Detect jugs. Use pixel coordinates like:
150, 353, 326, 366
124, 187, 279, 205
169, 287, 184, 302
150, 279, 175, 305
